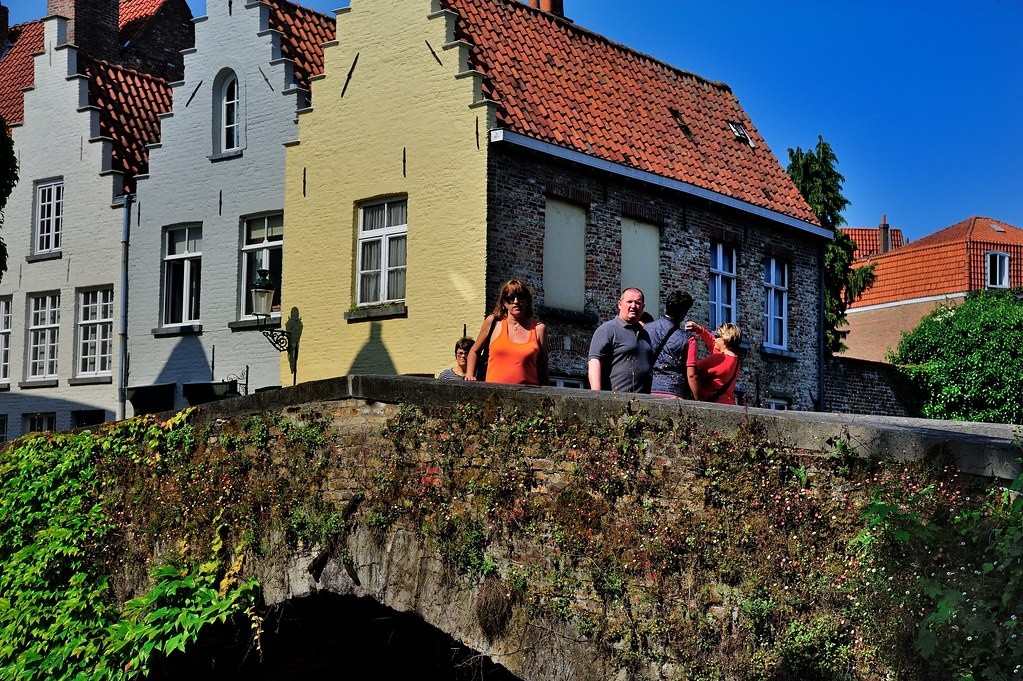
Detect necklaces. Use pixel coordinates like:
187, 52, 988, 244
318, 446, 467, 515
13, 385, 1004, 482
507, 318, 519, 330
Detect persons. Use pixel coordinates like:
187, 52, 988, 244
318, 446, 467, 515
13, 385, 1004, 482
684, 321, 742, 405
638, 290, 701, 401
587, 288, 654, 394
437, 338, 485, 381
465, 279, 550, 385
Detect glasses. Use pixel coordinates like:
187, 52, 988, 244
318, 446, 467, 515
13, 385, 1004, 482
714, 334, 721, 338
456, 352, 468, 355
503, 294, 527, 303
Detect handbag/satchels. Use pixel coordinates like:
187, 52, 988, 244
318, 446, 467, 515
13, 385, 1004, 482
474, 341, 489, 381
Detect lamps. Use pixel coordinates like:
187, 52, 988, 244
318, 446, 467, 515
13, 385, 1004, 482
249, 266, 293, 354
